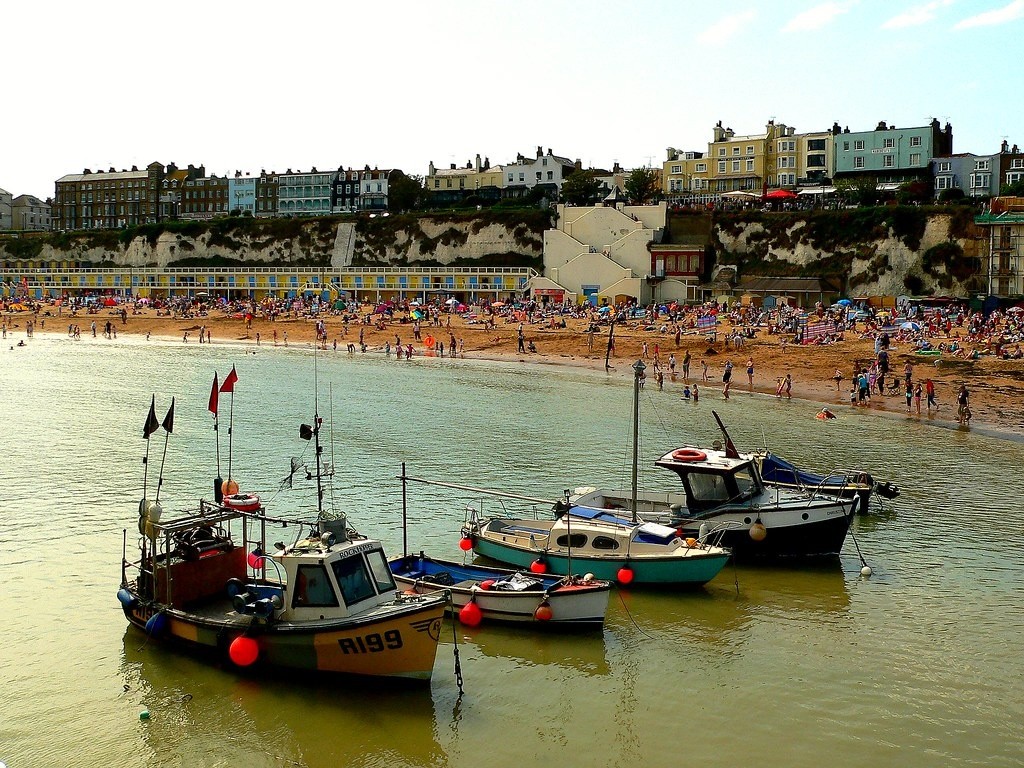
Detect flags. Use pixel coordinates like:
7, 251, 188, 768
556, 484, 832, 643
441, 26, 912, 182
143, 399, 160, 439
208, 373, 219, 416
160, 397, 174, 433
219, 370, 238, 393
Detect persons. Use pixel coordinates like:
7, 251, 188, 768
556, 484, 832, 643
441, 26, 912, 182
0, 276, 1024, 425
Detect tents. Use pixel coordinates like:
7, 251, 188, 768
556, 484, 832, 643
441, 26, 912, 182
330, 301, 346, 310
793, 314, 837, 342
900, 321, 919, 332
837, 299, 852, 310
875, 312, 890, 319
947, 313, 959, 326
407, 311, 422, 320
696, 314, 717, 334
456, 304, 468, 313
375, 301, 387, 313
510, 310, 527, 321
629, 308, 648, 320
102, 298, 118, 306
138, 297, 149, 305
385, 301, 396, 311
851, 310, 871, 321
6, 304, 29, 311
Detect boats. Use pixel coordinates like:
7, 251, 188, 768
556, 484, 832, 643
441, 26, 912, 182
555, 410, 901, 564
116, 331, 454, 688
461, 358, 743, 591
367, 461, 615, 633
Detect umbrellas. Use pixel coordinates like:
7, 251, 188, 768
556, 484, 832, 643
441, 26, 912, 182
196, 292, 208, 297
492, 301, 504, 307
1005, 307, 1023, 315
598, 307, 611, 313
446, 299, 459, 305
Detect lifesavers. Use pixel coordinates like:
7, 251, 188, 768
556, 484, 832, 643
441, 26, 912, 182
223, 493, 260, 511
424, 337, 434, 346
672, 449, 707, 460
